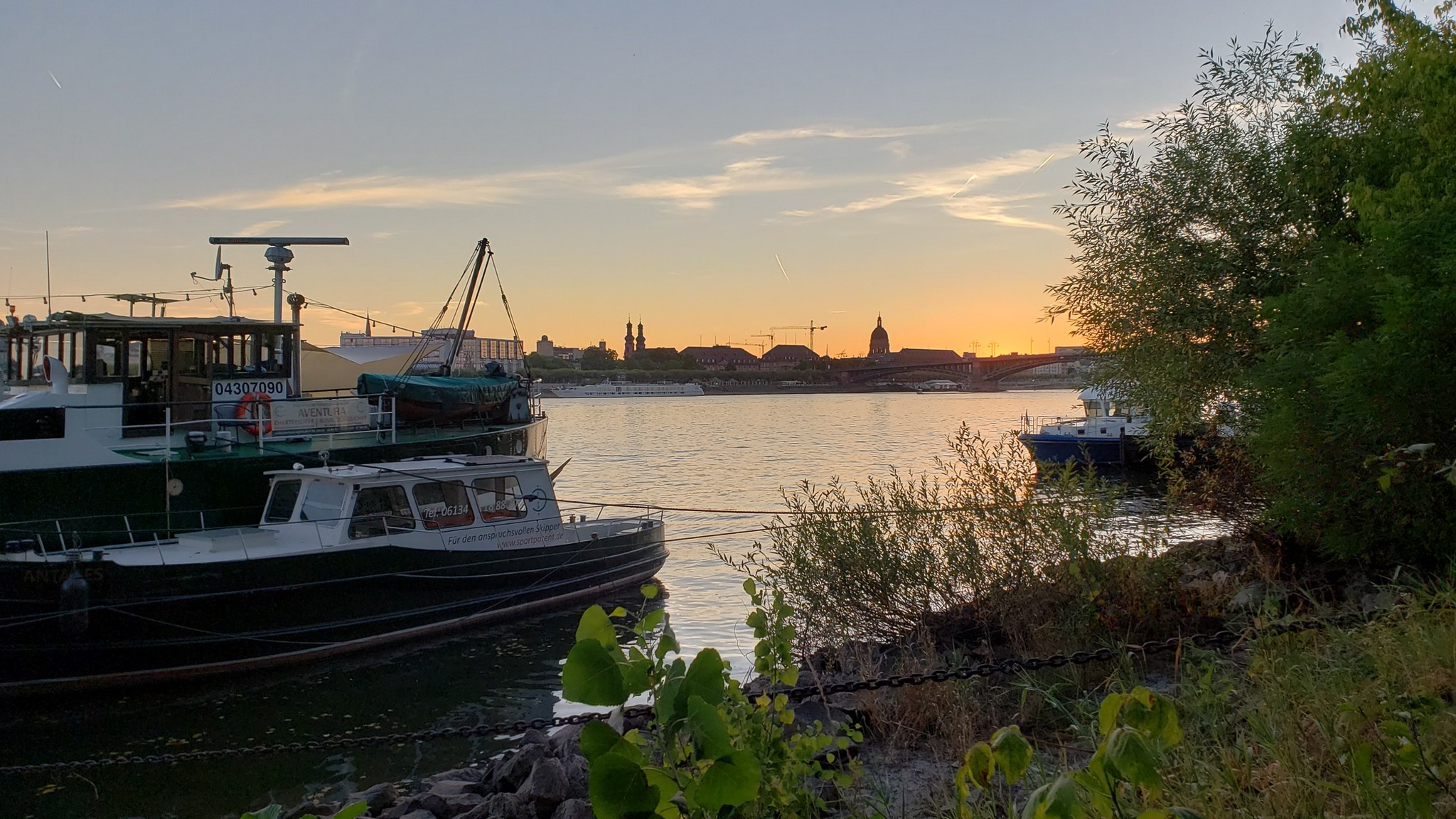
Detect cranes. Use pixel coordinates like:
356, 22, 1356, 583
726, 334, 774, 356
770, 320, 827, 351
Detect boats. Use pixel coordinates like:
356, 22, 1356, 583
0, 237, 669, 717
550, 377, 704, 398
1014, 384, 1235, 480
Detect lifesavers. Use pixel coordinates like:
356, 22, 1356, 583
235, 391, 272, 436
